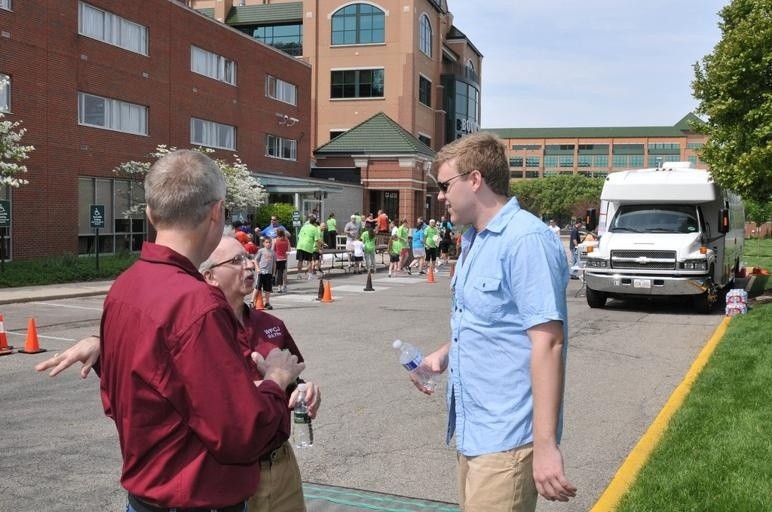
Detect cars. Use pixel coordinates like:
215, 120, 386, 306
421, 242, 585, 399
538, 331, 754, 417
565, 232, 600, 283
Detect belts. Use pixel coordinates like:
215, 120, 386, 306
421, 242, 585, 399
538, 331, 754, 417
260, 441, 288, 468
128, 494, 245, 512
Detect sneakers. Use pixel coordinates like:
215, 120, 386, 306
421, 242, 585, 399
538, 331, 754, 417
406, 265, 436, 274
571, 275, 579, 279
265, 303, 272, 309
278, 284, 287, 293
297, 269, 322, 279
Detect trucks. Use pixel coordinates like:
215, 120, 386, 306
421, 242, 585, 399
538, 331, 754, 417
583, 162, 754, 313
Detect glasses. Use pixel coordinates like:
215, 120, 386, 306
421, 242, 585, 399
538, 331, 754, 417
204, 199, 229, 221
438, 172, 468, 190
210, 254, 253, 268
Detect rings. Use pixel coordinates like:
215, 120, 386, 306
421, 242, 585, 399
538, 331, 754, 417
54, 353, 59, 357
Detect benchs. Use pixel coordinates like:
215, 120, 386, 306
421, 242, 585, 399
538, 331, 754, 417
374, 244, 388, 264
319, 248, 354, 270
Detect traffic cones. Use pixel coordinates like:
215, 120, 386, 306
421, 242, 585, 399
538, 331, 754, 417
426, 264, 436, 285
255, 289, 269, 315
364, 270, 376, 290
323, 280, 334, 305
450, 263, 456, 277
318, 279, 326, 300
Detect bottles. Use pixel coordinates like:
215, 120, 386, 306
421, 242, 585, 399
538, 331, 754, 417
392, 339, 443, 392
291, 383, 314, 449
725, 288, 748, 316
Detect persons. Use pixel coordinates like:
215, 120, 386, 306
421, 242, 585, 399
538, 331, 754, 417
570, 218, 582, 280
100, 148, 306, 512
231, 210, 463, 310
410, 131, 577, 512
547, 219, 560, 235
35, 234, 322, 512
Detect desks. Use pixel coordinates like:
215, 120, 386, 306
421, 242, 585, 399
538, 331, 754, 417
335, 234, 347, 249
